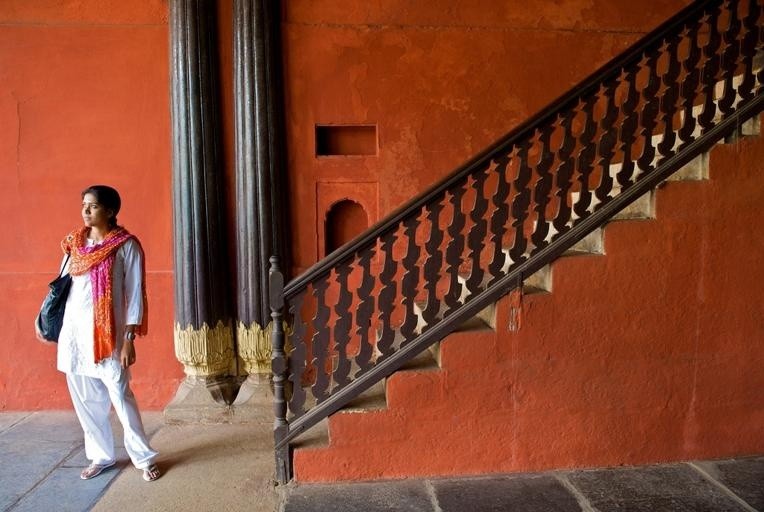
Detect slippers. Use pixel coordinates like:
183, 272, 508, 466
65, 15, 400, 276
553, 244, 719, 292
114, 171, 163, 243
141, 465, 162, 482
81, 459, 116, 480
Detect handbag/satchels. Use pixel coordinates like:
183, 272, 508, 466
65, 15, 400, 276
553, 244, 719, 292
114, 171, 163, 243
34, 252, 74, 343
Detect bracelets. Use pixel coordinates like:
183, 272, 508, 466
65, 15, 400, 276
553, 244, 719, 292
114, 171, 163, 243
124, 332, 136, 341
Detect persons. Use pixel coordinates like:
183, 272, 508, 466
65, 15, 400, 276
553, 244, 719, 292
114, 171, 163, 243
36, 187, 162, 483
